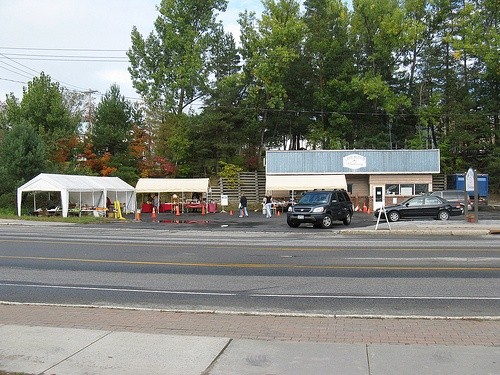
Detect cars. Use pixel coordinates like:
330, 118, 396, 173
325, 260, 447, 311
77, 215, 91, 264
374, 191, 462, 222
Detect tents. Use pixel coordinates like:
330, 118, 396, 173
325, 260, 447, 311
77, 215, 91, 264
135, 178, 213, 214
17, 173, 137, 218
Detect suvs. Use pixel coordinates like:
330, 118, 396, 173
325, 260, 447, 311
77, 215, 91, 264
429, 190, 472, 215
285, 189, 355, 229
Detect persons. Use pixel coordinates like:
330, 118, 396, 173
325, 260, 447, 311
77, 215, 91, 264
106, 191, 274, 219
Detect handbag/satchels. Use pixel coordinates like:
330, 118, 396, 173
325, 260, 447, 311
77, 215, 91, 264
238, 203, 242, 208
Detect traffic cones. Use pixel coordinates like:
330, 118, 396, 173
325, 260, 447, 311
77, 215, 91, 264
135, 212, 142, 222
175, 206, 181, 216
151, 207, 157, 218
363, 202, 367, 213
242, 210, 244, 215
276, 211, 280, 216
201, 205, 205, 214
229, 209, 233, 216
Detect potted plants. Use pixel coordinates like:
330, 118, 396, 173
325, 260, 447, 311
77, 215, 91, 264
466, 213, 475, 223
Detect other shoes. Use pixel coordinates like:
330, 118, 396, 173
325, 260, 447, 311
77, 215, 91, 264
239, 215, 243, 218
